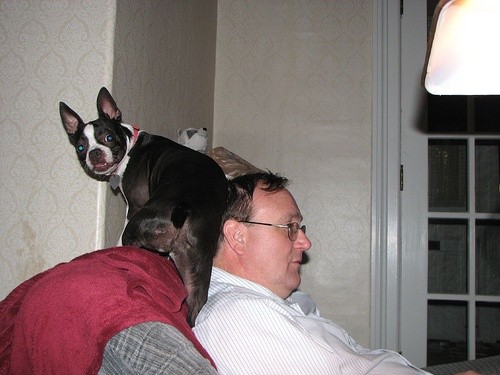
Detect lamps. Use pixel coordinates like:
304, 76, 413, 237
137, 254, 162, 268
420, 0, 498, 95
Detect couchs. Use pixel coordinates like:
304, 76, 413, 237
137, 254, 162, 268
0, 247, 499, 375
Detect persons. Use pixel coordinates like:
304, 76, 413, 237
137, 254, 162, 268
190, 168, 476, 374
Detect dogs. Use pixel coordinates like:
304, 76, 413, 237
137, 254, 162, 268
59, 88, 227, 329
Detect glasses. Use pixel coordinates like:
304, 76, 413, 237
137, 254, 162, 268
221, 220, 307, 242
